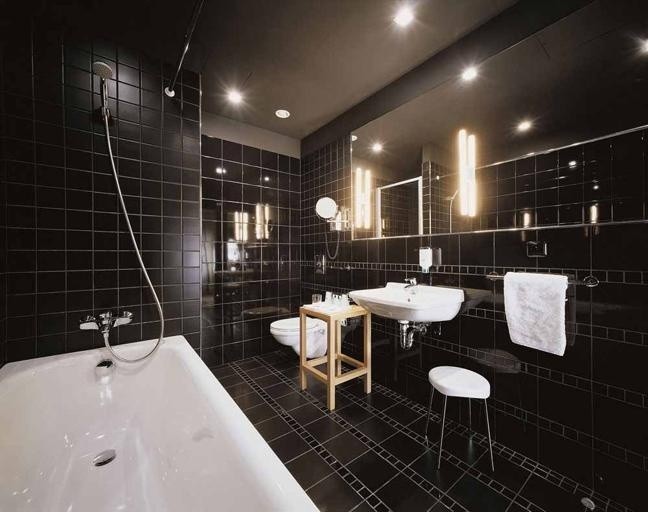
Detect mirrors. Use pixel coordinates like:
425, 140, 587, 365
350, 0, 648, 241
316, 196, 351, 223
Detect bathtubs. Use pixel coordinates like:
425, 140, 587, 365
0, 335, 320, 512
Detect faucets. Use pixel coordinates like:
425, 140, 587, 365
235, 263, 245, 271
98, 312, 112, 333
404, 277, 416, 289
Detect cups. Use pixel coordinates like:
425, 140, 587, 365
312, 294, 322, 307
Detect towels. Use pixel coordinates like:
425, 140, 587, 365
565, 274, 577, 346
503, 272, 568, 357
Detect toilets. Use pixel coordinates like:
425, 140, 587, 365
242, 307, 290, 328
270, 317, 327, 358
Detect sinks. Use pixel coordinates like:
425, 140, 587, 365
348, 283, 465, 322
214, 269, 254, 281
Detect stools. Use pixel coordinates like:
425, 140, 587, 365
458, 348, 526, 432
299, 305, 371, 411
425, 366, 494, 473
